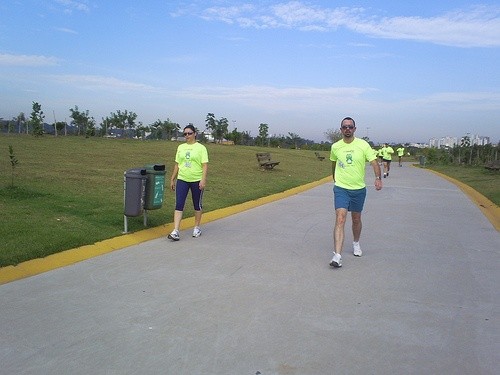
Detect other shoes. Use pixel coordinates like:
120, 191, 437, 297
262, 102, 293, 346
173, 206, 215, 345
168, 229, 180, 240
330, 251, 343, 267
192, 228, 201, 237
353, 242, 362, 256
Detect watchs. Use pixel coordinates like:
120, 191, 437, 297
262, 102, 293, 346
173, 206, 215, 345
377, 177, 381, 179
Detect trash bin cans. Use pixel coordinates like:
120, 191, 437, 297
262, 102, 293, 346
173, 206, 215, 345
145, 164, 167, 210
125, 168, 148, 217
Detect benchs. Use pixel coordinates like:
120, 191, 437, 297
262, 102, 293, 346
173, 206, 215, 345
314, 152, 325, 161
256, 152, 281, 170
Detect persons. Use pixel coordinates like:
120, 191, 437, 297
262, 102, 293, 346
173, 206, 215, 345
169, 125, 208, 241
397, 145, 405, 167
372, 143, 394, 178
329, 117, 381, 268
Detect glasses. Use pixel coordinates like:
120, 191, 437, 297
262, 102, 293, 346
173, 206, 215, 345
184, 132, 192, 135
342, 126, 354, 130
